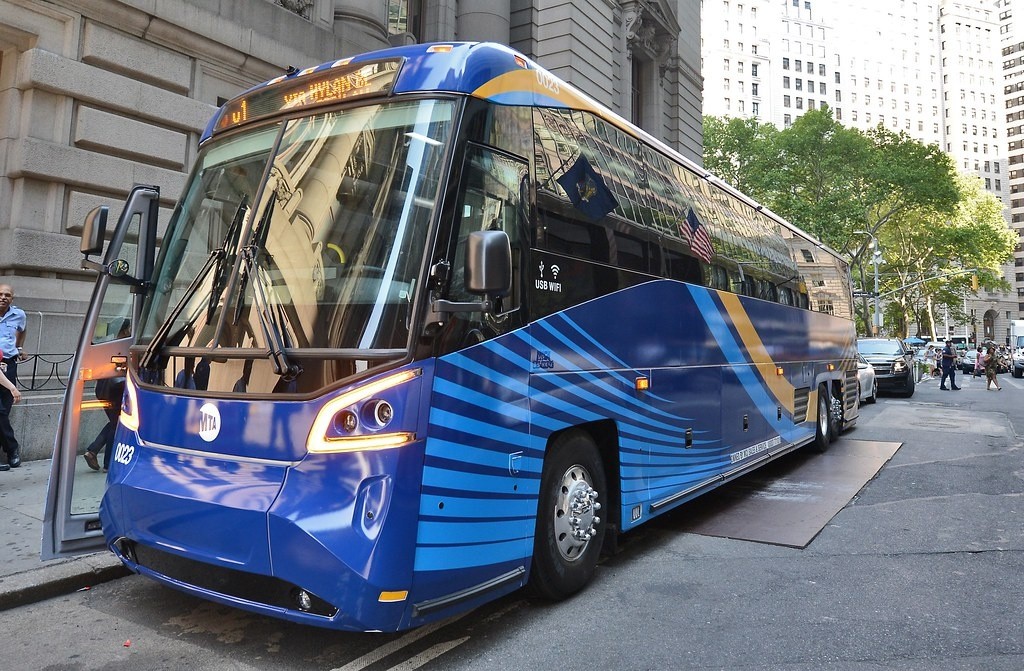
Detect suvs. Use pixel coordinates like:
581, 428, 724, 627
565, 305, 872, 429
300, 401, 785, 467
857, 337, 915, 397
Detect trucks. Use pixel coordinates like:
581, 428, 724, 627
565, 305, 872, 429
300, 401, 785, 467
1005, 320, 1024, 377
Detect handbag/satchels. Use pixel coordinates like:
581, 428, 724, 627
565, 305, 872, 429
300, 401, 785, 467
979, 356, 989, 367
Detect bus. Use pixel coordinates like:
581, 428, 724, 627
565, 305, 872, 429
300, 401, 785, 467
39, 41, 861, 633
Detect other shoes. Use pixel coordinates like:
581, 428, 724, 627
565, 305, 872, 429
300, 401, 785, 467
102, 466, 107, 473
84, 450, 100, 470
0, 462, 10, 471
941, 386, 950, 390
952, 385, 961, 390
8, 447, 20, 467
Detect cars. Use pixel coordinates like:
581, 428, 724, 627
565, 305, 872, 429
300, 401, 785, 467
857, 351, 878, 407
956, 350, 966, 370
962, 349, 987, 374
911, 348, 933, 364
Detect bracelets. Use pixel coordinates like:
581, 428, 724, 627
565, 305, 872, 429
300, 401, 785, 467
17, 346, 22, 349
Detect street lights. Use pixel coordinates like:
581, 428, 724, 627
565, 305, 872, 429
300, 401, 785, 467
852, 231, 989, 337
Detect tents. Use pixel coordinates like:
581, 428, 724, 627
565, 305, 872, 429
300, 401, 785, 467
903, 336, 925, 343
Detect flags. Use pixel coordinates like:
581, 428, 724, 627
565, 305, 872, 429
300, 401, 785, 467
556, 152, 619, 223
679, 206, 715, 264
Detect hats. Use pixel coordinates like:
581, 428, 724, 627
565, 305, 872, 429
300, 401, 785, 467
946, 340, 953, 344
110, 317, 130, 331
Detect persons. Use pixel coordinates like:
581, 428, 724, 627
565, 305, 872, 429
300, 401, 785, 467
924, 340, 1007, 390
84, 317, 131, 473
0, 284, 28, 471
